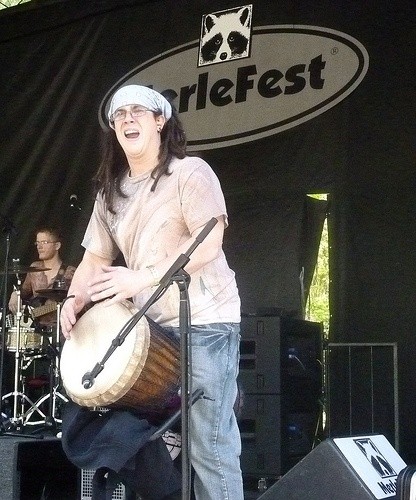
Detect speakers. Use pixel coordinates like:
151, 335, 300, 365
232, 317, 408, 500
80, 468, 127, 500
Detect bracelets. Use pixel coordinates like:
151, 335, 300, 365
146, 265, 162, 288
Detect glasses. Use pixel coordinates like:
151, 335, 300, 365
110, 107, 154, 121
34, 241, 56, 245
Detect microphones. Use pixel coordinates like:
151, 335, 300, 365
70, 194, 77, 208
82, 372, 95, 388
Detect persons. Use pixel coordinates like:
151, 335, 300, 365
60, 84, 244, 500
8, 226, 76, 354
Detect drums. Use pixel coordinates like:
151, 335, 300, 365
58, 297, 188, 416
0, 327, 51, 353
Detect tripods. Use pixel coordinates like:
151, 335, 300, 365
2, 274, 69, 434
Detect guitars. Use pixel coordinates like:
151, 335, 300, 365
6, 302, 61, 328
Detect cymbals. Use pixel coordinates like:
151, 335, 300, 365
36, 287, 68, 299
0, 265, 52, 275
